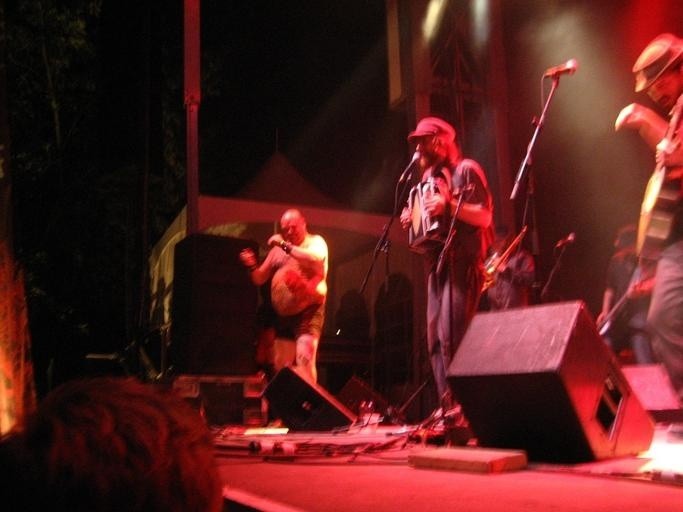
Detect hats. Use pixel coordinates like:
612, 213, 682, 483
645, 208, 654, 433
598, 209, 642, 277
405, 116, 456, 146
632, 32, 683, 94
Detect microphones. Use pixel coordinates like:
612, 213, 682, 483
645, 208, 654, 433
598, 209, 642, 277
398, 152, 421, 184
557, 232, 575, 247
545, 59, 577, 78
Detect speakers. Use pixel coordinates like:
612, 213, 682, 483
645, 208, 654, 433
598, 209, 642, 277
171, 235, 259, 374
445, 301, 655, 465
262, 366, 357, 430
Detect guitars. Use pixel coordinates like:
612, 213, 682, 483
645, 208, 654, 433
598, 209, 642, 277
636, 94, 683, 263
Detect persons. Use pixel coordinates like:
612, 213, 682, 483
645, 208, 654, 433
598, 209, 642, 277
401, 115, 498, 448
238, 207, 331, 387
612, 36, 683, 393
487, 221, 537, 310
595, 224, 656, 365
0, 373, 230, 511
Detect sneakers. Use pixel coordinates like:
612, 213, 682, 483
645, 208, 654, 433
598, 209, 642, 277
406, 404, 477, 446
